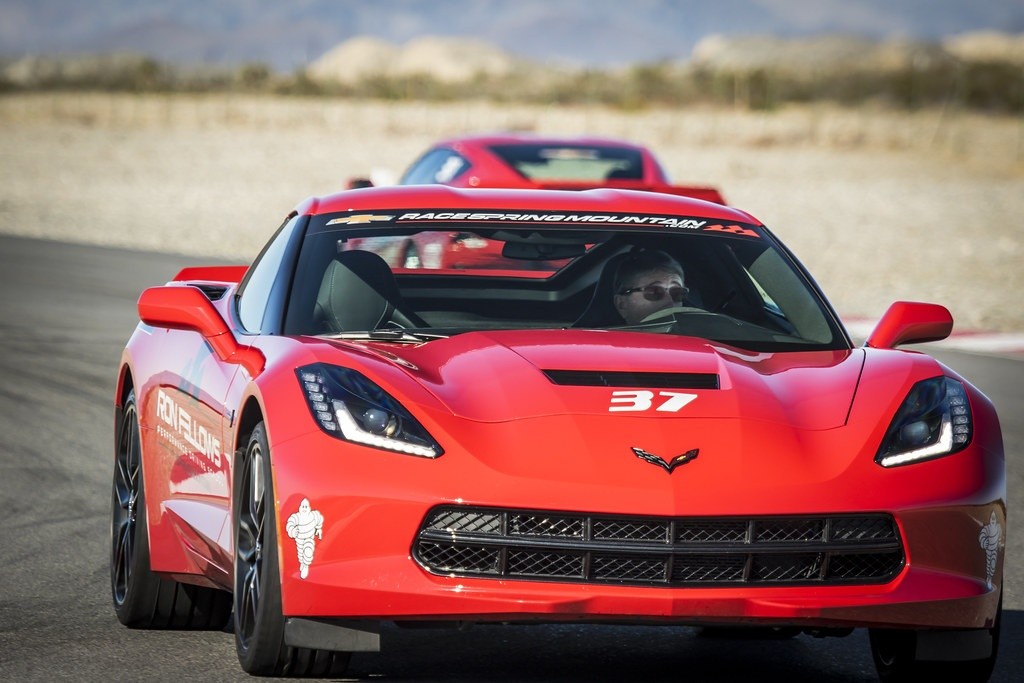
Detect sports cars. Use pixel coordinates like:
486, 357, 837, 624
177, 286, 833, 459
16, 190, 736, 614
104, 184, 1005, 678
346, 138, 725, 287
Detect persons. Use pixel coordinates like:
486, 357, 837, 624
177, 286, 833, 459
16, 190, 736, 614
616, 250, 688, 327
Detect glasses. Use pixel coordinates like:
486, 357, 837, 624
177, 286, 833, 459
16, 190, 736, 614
617, 282, 691, 303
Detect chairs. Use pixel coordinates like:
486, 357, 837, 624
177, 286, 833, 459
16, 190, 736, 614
571, 255, 627, 328
314, 247, 431, 335
